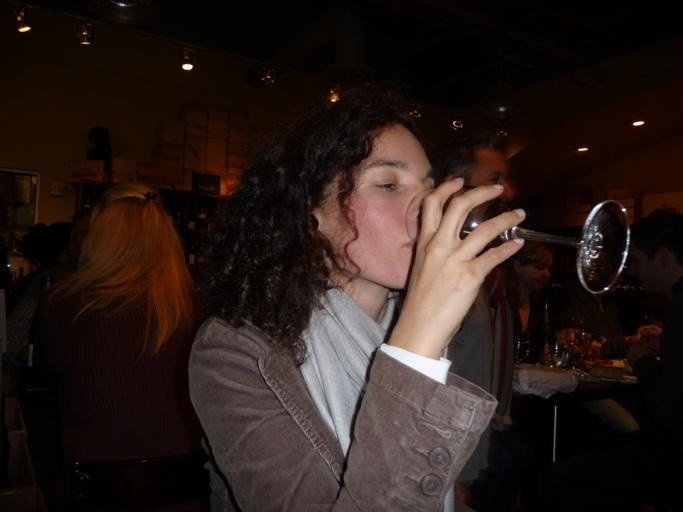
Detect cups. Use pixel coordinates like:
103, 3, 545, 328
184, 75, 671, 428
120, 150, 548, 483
550, 343, 573, 368
596, 365, 627, 382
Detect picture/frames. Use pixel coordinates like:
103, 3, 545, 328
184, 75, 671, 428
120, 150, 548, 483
0, 167, 41, 256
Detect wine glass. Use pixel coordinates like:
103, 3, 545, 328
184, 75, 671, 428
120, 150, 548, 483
403, 185, 630, 296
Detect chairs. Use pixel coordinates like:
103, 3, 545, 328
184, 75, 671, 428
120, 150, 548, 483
62, 450, 210, 511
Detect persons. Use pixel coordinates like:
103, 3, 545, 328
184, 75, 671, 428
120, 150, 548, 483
560, 275, 652, 435
511, 241, 557, 362
184, 89, 529, 512
25, 180, 215, 512
7, 205, 94, 372
401, 127, 522, 507
591, 200, 683, 512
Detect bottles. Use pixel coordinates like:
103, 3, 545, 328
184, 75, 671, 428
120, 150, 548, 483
40, 270, 57, 290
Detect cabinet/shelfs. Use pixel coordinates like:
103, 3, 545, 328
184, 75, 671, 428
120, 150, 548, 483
75, 180, 222, 277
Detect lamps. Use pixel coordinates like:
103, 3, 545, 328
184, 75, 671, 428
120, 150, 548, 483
9, 0, 280, 89
